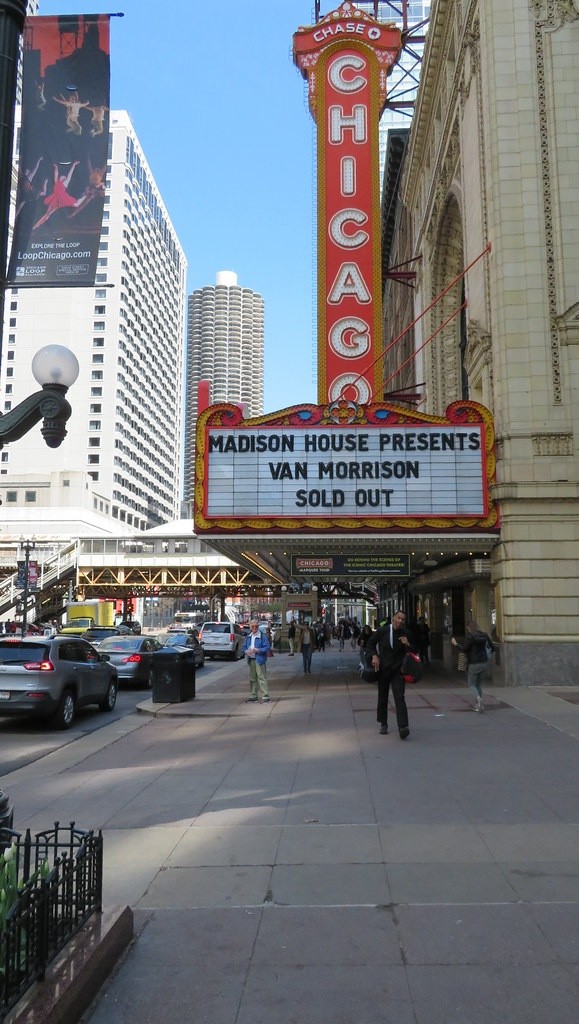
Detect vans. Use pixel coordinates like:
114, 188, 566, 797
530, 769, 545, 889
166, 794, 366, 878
199, 622, 247, 660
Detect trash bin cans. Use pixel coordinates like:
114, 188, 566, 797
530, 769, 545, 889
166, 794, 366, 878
152, 646, 195, 703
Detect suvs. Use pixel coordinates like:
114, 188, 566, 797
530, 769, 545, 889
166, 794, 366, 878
0, 635, 118, 730
120, 621, 141, 634
82, 625, 134, 650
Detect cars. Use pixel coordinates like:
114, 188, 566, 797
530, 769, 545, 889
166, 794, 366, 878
154, 633, 207, 668
168, 628, 199, 633
96, 636, 163, 690
244, 621, 282, 645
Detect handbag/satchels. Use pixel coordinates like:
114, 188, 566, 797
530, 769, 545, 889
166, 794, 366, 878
356, 645, 378, 683
458, 652, 468, 671
401, 652, 424, 684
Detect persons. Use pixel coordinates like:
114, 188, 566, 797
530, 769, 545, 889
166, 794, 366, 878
131, 547, 136, 553
148, 625, 154, 631
162, 546, 168, 552
299, 621, 314, 673
5, 618, 16, 633
310, 614, 363, 652
451, 621, 495, 711
287, 620, 296, 656
415, 617, 431, 663
45, 620, 63, 634
366, 610, 411, 740
16, 78, 109, 230
357, 625, 374, 648
242, 619, 271, 704
34, 566, 51, 578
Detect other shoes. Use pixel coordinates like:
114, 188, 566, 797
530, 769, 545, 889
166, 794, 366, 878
380, 722, 388, 734
288, 654, 294, 656
262, 699, 271, 705
472, 696, 484, 714
245, 698, 259, 703
399, 727, 410, 740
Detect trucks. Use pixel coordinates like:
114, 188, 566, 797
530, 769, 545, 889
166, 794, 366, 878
59, 602, 114, 636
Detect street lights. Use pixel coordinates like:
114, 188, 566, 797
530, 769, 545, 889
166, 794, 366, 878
20, 534, 37, 635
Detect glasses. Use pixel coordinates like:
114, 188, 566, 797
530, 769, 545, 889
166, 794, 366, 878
252, 626, 258, 627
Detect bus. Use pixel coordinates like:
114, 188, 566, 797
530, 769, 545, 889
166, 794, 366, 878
174, 610, 203, 628
